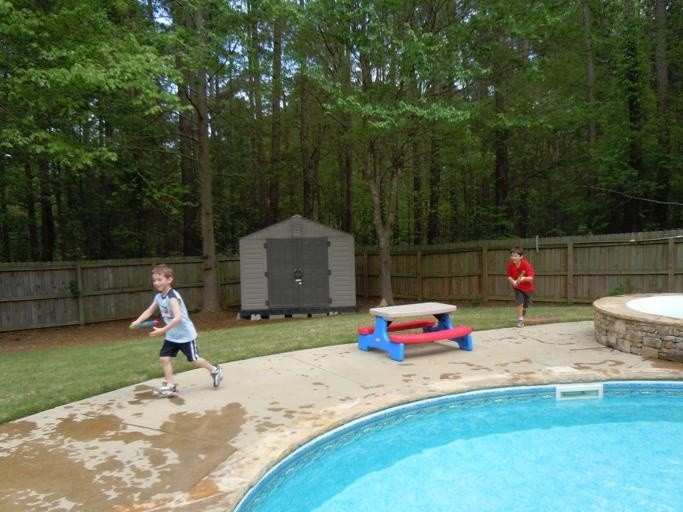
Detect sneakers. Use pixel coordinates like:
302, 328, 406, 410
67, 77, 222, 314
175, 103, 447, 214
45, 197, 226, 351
517, 317, 524, 327
154, 384, 177, 397
210, 363, 223, 387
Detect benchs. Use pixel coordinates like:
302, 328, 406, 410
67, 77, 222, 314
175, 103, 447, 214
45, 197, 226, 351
357, 319, 436, 335
388, 325, 471, 344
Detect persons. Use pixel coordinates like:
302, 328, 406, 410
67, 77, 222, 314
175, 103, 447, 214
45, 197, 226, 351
130, 263, 223, 395
507, 246, 535, 328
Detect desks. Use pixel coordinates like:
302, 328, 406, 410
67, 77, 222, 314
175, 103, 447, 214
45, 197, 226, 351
358, 301, 472, 362
